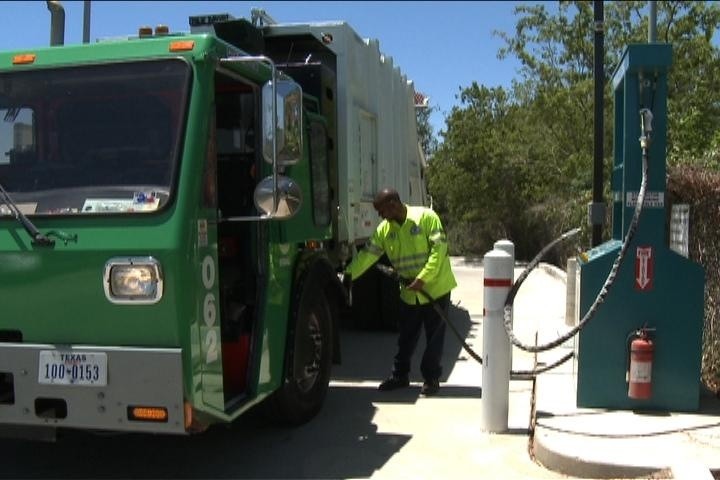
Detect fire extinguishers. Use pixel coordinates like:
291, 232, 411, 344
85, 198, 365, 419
625, 321, 657, 400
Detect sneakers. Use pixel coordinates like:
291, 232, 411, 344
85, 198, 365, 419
421, 375, 441, 397
378, 371, 411, 392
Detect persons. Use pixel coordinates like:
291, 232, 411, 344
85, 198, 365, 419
339, 184, 459, 397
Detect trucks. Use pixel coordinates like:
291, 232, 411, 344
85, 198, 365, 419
0, 1, 435, 442
